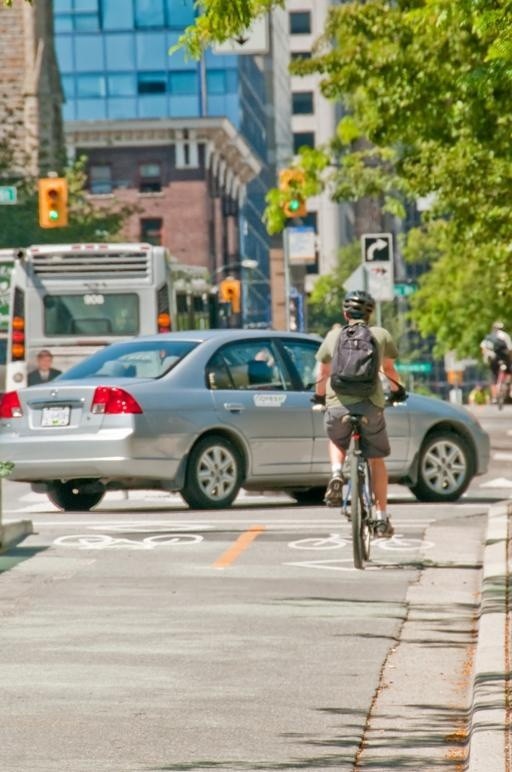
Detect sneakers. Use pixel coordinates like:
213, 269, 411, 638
375, 520, 394, 537
325, 470, 343, 506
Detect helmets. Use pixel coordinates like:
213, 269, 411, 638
344, 290, 375, 318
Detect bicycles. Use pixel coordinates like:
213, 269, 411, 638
310, 391, 409, 569
495, 349, 511, 410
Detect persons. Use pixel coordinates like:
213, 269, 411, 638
248, 348, 275, 384
310, 290, 406, 537
27, 350, 61, 386
468, 321, 512, 404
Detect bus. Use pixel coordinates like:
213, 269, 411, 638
5, 242, 177, 400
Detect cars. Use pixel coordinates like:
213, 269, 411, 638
0, 328, 488, 510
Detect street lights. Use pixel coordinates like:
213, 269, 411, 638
200, 259, 258, 330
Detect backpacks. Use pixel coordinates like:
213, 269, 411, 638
330, 322, 378, 397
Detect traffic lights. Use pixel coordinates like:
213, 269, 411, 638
219, 281, 239, 314
37, 176, 72, 228
282, 169, 305, 218
